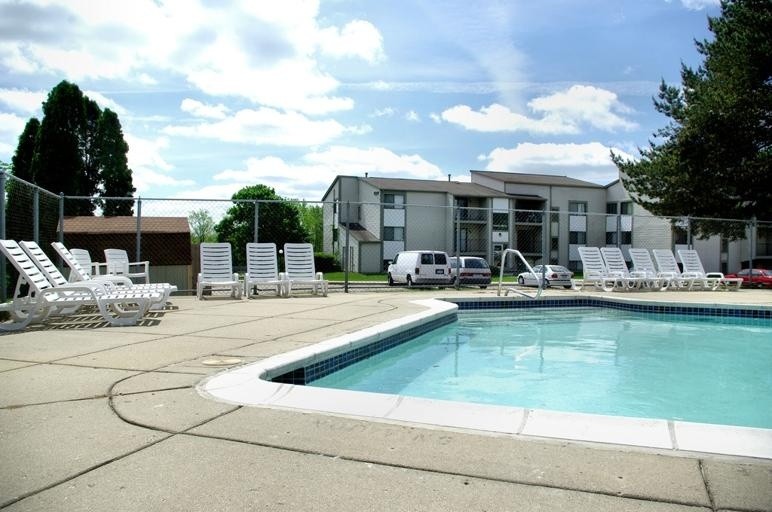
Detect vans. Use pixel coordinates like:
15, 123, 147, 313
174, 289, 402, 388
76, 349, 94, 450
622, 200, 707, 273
449, 256, 492, 289
386, 249, 453, 291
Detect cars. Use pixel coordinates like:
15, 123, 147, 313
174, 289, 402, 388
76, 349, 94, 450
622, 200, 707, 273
516, 263, 574, 289
724, 267, 772, 287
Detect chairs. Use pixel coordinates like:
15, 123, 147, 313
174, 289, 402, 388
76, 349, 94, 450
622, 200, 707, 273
577, 246, 744, 292
244, 241, 286, 297
284, 243, 329, 297
1, 238, 177, 332
196, 243, 242, 300
